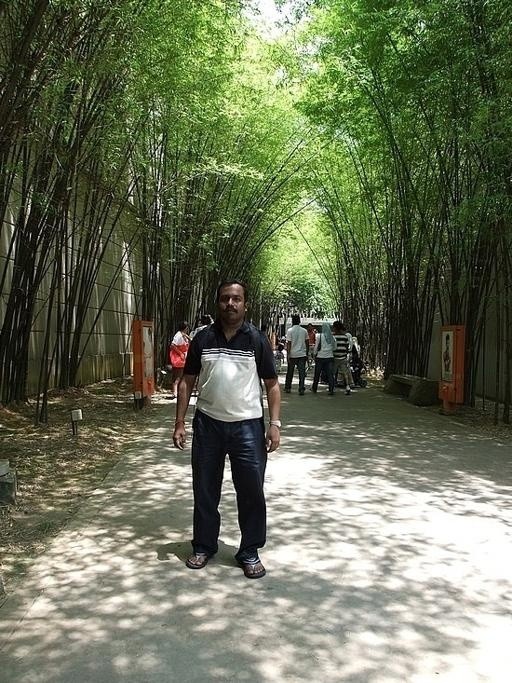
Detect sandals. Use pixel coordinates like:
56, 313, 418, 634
240, 554, 266, 579
185, 550, 214, 569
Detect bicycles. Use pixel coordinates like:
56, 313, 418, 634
305, 344, 317, 376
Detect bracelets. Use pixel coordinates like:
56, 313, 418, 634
175, 421, 186, 424
268, 420, 280, 427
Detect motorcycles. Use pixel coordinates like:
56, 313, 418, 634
277, 335, 287, 351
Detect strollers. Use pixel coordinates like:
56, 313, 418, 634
348, 357, 369, 388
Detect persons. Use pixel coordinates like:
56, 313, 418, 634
173, 281, 281, 579
170, 314, 215, 397
443, 334, 452, 374
283, 315, 356, 395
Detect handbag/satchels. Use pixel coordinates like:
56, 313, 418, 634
316, 333, 322, 352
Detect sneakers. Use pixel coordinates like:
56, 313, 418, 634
284, 384, 364, 395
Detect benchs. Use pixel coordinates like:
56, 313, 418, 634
383, 372, 441, 404
